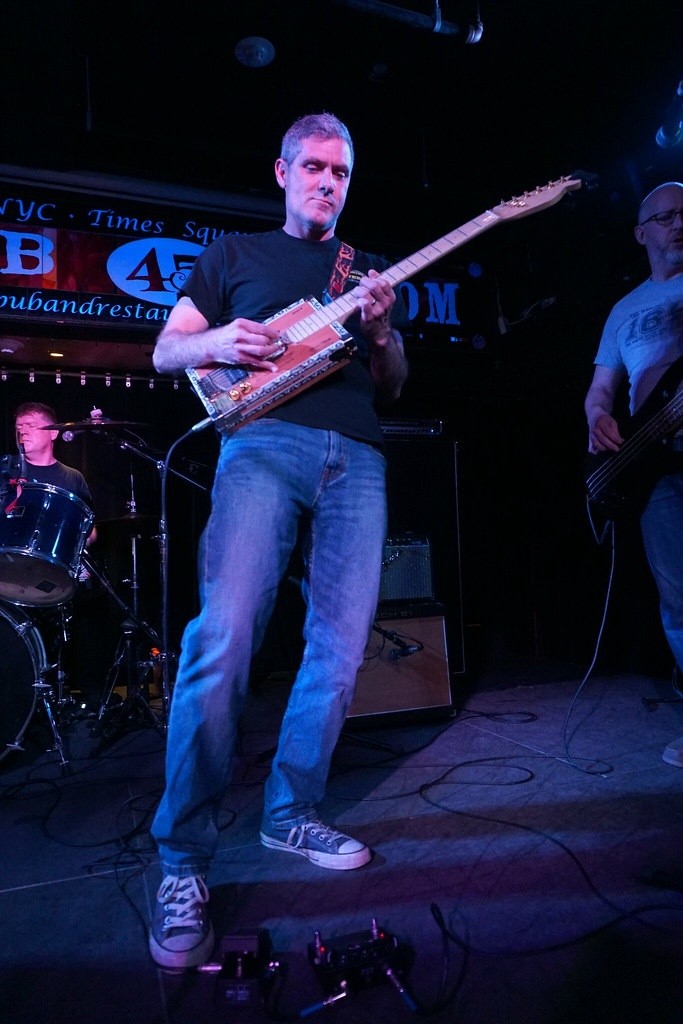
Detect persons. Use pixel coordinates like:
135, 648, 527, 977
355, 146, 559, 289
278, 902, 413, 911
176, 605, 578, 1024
0, 403, 98, 545
579, 182, 683, 769
151, 111, 410, 968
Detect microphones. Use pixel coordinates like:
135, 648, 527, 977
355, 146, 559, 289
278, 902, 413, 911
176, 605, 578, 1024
18, 443, 27, 479
389, 645, 420, 660
61, 429, 84, 441
656, 82, 683, 149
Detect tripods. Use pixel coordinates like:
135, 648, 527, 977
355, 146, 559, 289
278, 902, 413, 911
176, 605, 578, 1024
86, 427, 209, 738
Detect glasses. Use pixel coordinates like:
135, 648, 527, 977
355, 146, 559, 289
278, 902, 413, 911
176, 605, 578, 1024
640, 208, 683, 226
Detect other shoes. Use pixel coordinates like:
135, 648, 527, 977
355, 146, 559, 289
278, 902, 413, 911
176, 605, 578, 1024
662, 736, 683, 767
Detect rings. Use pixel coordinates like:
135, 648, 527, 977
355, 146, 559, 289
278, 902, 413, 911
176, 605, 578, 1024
371, 299, 376, 306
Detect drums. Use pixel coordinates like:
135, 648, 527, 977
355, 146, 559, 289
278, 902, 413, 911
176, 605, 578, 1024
0, 600, 49, 763
0, 480, 97, 609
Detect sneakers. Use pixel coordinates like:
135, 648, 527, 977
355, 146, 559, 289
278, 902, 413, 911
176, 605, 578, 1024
150, 871, 213, 967
260, 818, 370, 871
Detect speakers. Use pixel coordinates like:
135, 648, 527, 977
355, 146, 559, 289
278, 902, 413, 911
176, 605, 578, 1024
341, 433, 466, 730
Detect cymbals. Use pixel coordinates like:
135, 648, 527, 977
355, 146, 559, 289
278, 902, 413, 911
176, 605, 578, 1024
36, 406, 153, 432
97, 509, 161, 523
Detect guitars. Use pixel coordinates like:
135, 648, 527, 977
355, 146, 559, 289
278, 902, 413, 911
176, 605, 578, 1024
184, 169, 584, 439
584, 354, 683, 526
